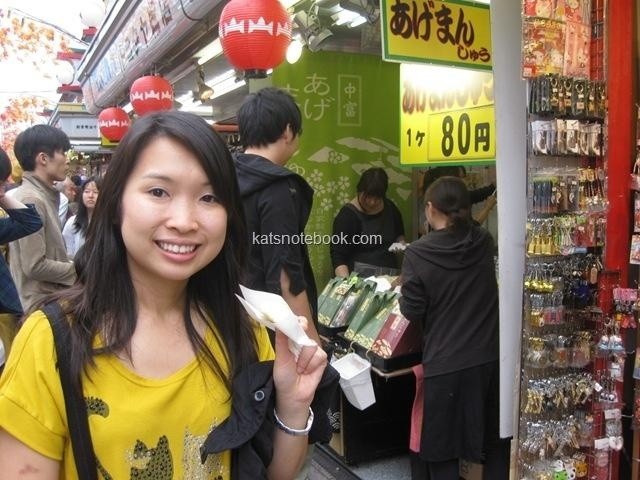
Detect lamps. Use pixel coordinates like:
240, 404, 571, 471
192, 57, 215, 102
293, 1, 334, 54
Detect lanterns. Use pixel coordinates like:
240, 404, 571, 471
129, 75, 174, 115
97, 106, 132, 142
218, 1, 291, 79
